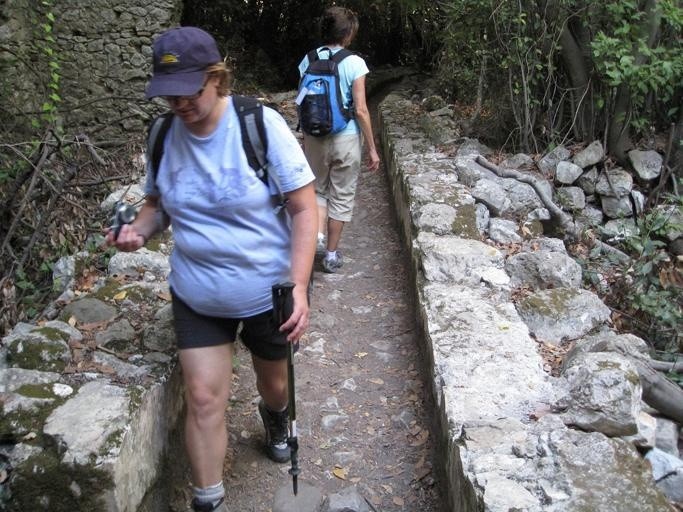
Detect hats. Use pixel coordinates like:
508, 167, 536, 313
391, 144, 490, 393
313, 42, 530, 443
145, 26, 222, 101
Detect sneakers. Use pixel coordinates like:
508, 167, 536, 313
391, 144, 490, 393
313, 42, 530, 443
192, 494, 228, 511
316, 239, 344, 273
258, 397, 292, 462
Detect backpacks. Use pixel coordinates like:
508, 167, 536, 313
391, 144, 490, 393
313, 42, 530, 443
298, 48, 358, 138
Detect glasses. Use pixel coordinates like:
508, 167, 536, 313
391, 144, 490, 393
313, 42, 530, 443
159, 72, 214, 101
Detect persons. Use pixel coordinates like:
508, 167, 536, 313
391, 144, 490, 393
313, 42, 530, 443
102, 25, 323, 512
299, 6, 380, 273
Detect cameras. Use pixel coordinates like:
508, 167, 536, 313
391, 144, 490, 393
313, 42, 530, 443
108, 200, 137, 241
